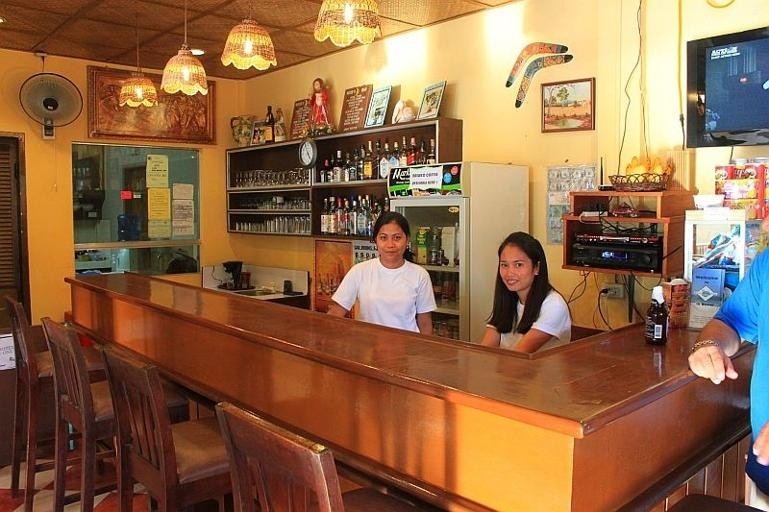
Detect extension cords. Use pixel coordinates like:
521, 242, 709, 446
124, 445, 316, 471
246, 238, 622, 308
582, 211, 608, 216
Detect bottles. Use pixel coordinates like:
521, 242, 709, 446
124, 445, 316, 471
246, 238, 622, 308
329, 197, 337, 236
415, 227, 428, 263
228, 191, 311, 235
321, 198, 329, 234
431, 271, 460, 340
365, 141, 372, 177
320, 158, 329, 182
73, 166, 103, 221
343, 146, 359, 182
425, 228, 440, 264
342, 200, 351, 235
263, 106, 274, 145
359, 145, 364, 179
357, 194, 366, 237
76, 250, 106, 261
647, 286, 669, 343
349, 201, 356, 234
337, 198, 343, 236
328, 160, 334, 181
333, 150, 342, 181
363, 195, 389, 234
373, 139, 381, 178
360, 198, 368, 234
382, 137, 436, 177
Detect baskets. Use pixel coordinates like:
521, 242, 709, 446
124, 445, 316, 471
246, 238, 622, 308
608, 173, 669, 191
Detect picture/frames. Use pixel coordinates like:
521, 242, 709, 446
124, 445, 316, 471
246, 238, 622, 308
363, 84, 392, 128
249, 120, 265, 146
540, 78, 596, 133
86, 64, 216, 145
416, 81, 446, 120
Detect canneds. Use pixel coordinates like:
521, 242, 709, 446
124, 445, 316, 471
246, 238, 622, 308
429, 250, 444, 266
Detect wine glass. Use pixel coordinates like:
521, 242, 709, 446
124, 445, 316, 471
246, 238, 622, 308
234, 168, 310, 185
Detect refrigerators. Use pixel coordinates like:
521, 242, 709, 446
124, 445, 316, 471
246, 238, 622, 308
386, 161, 528, 346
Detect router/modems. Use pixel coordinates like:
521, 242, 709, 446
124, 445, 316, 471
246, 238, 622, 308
598, 156, 616, 191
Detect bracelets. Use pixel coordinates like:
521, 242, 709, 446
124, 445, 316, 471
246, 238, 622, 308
687, 339, 722, 354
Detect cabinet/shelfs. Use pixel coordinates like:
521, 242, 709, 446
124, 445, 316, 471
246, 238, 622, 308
314, 117, 462, 240
562, 190, 691, 278
72, 157, 105, 219
226, 139, 313, 237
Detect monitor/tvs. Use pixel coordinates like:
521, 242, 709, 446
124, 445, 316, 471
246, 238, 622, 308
687, 26, 769, 148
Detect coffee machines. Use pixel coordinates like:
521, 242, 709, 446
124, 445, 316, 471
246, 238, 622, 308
222, 261, 242, 290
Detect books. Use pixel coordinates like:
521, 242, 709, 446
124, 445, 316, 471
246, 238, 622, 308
691, 267, 724, 331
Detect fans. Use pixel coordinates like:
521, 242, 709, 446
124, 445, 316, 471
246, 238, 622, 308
16, 74, 84, 139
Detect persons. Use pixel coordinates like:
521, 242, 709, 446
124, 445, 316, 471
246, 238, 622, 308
264, 105, 274, 124
688, 215, 769, 512
271, 106, 286, 135
259, 131, 267, 144
327, 212, 437, 338
252, 132, 260, 143
308, 78, 335, 135
480, 231, 572, 354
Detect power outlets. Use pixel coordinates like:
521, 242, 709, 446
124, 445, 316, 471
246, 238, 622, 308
600, 282, 625, 298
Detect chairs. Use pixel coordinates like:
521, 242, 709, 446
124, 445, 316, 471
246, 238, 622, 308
214, 400, 438, 512
98, 341, 257, 512
0, 290, 110, 512
40, 317, 190, 512
665, 493, 767, 512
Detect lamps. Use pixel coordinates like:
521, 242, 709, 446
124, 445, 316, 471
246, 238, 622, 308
314, 0, 383, 47
160, 0, 209, 96
220, 1, 277, 71
118, 11, 159, 107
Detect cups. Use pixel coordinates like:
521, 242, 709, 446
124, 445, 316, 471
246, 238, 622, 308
240, 272, 251, 290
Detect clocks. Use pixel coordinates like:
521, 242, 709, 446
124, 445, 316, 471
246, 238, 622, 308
298, 137, 318, 168
